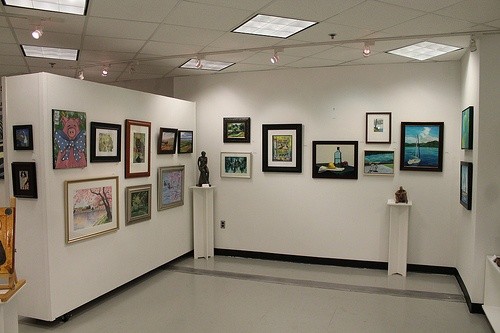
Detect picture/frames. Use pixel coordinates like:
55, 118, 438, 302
312, 141, 358, 180
461, 106, 474, 149
51, 109, 194, 244
366, 112, 391, 144
459, 161, 473, 210
12, 124, 33, 150
363, 149, 394, 176
11, 162, 38, 199
400, 121, 444, 172
223, 118, 250, 142
220, 152, 251, 179
262, 124, 302, 173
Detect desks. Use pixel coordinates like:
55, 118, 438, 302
482, 256, 500, 333
387, 199, 412, 277
0, 281, 26, 333
189, 186, 216, 260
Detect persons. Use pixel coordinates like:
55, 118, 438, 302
196, 151, 212, 187
395, 185, 408, 204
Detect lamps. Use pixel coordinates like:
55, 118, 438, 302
469, 34, 477, 53
271, 47, 284, 64
363, 41, 376, 57
76, 68, 84, 80
31, 24, 43, 40
101, 64, 111, 76
129, 60, 140, 72
195, 56, 206, 71
